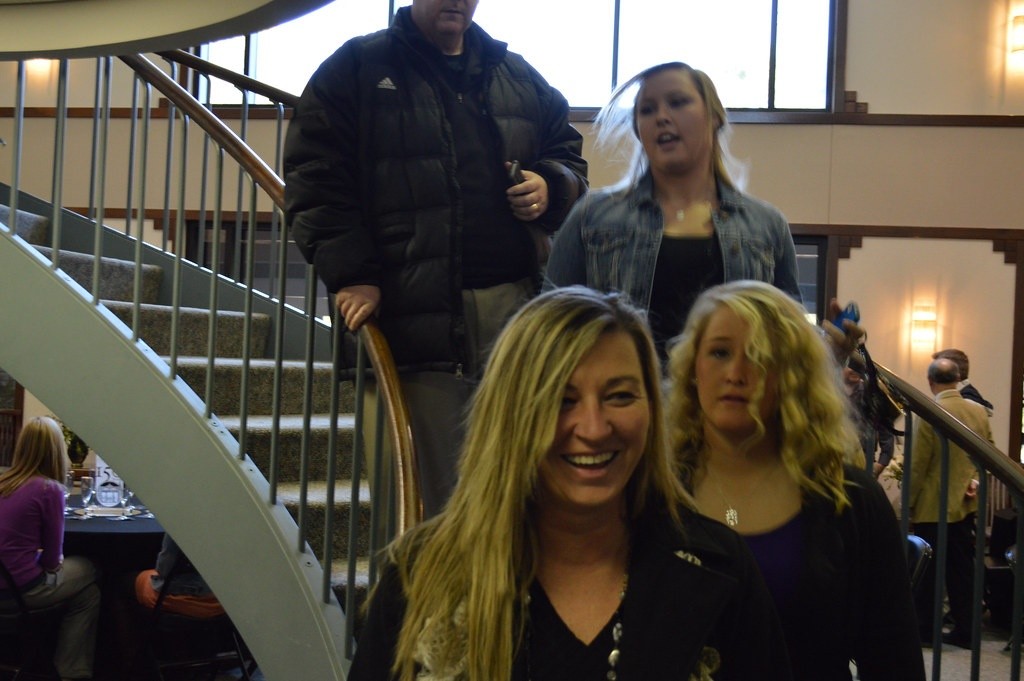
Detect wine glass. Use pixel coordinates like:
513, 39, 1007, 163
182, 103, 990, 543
78, 476, 93, 518
127, 488, 135, 510
117, 479, 129, 520
63, 475, 72, 515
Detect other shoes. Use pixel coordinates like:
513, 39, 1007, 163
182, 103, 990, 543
942, 630, 973, 650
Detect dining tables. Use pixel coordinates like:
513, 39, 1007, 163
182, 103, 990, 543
63, 493, 166, 537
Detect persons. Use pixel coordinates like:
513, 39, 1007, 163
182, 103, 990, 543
0, 415, 104, 680
659, 278, 929, 681
841, 366, 894, 479
341, 284, 793, 681
283, 0, 590, 531
535, 61, 802, 380
931, 349, 994, 418
112, 531, 215, 681
901, 358, 996, 651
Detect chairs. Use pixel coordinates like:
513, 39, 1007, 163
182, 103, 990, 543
907, 534, 933, 592
119, 553, 252, 681
0, 561, 63, 681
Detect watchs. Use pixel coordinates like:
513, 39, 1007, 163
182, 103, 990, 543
971, 477, 980, 486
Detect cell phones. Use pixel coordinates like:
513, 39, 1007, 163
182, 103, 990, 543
830, 300, 860, 336
508, 159, 531, 196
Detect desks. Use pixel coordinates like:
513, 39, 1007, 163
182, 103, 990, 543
985, 547, 1013, 570
